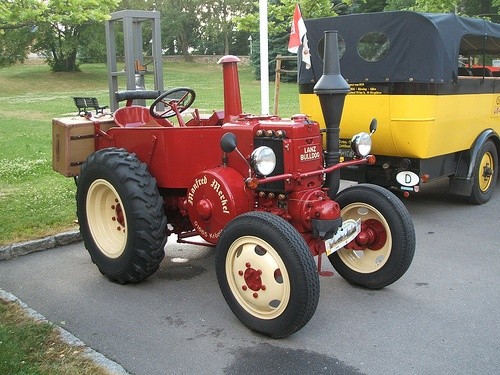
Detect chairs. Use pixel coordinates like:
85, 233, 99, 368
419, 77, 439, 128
113, 89, 174, 127
457, 66, 494, 77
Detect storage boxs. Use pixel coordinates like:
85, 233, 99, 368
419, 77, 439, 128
52, 115, 118, 178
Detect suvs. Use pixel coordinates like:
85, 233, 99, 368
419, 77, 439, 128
297, 10, 500, 206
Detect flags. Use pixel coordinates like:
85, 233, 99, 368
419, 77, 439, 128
288, 4, 307, 53
